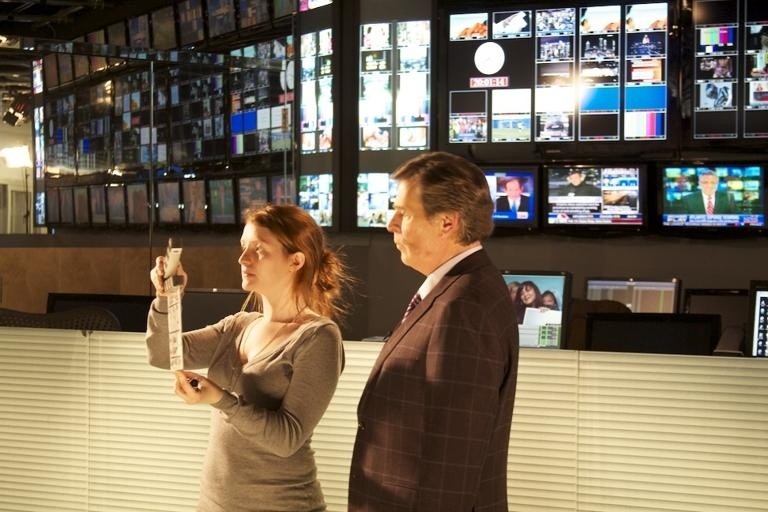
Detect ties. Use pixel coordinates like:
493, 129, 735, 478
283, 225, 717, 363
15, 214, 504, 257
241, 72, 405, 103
402, 294, 422, 323
707, 196, 713, 215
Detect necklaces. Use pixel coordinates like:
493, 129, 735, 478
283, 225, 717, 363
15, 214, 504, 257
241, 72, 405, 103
225, 303, 306, 394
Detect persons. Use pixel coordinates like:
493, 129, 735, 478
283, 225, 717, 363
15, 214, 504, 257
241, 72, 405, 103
559, 171, 600, 196
450, 117, 487, 142
672, 171, 739, 215
250, 180, 266, 200
145, 202, 346, 511
211, 181, 233, 215
496, 178, 529, 211
750, 26, 768, 78
705, 85, 731, 109
506, 279, 560, 324
348, 152, 520, 511
700, 56, 733, 79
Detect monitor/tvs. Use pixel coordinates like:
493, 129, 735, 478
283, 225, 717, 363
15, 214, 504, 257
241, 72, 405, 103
692, 0, 768, 140
448, 3, 669, 145
297, 173, 334, 229
271, 175, 297, 208
746, 280, 767, 358
107, 183, 128, 229
46, 186, 60, 224
180, 178, 209, 228
236, 176, 268, 227
59, 186, 75, 225
499, 269, 570, 349
298, 0, 334, 14
297, 26, 336, 154
585, 278, 682, 312
0, 0, 295, 176
356, 172, 398, 228
35, 191, 46, 225
359, 18, 431, 150
475, 164, 538, 230
88, 183, 108, 230
541, 157, 649, 233
207, 179, 237, 229
154, 178, 182, 230
73, 185, 91, 227
658, 158, 768, 236
125, 183, 150, 228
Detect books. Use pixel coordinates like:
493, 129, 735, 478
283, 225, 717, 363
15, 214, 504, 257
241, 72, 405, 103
518, 308, 562, 347
